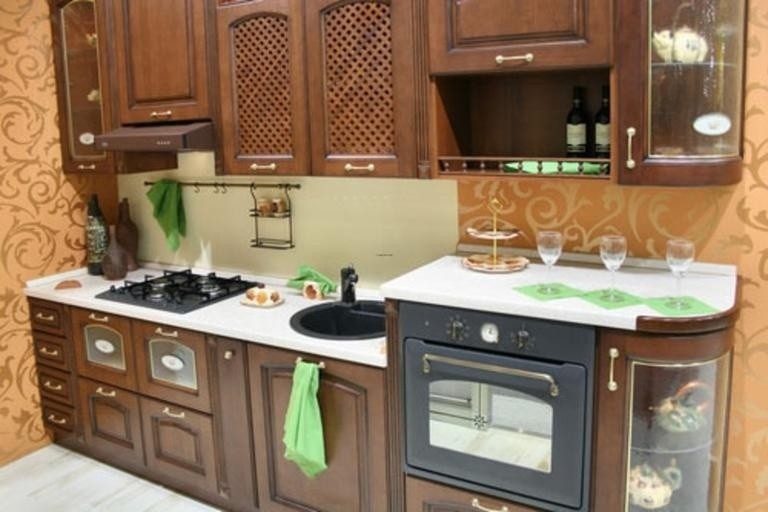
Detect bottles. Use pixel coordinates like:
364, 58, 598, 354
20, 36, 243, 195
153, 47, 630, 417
593, 84, 613, 158
115, 199, 140, 271
84, 192, 108, 277
563, 84, 589, 157
101, 223, 128, 280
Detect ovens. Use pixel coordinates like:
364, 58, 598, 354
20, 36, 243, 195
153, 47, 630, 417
395, 298, 594, 512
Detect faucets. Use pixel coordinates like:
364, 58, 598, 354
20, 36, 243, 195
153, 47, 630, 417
340, 267, 358, 304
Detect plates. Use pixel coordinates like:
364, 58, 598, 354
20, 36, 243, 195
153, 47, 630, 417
240, 293, 283, 307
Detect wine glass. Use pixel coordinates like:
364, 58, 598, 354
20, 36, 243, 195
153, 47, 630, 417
666, 238, 698, 310
534, 228, 563, 299
597, 235, 626, 302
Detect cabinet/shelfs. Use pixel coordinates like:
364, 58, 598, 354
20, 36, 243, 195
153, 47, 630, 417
23, 244, 749, 511
47, 0, 750, 188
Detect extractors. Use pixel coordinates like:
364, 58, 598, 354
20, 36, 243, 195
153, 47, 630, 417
93, 121, 214, 155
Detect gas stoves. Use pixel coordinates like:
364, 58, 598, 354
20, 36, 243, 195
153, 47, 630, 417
92, 265, 267, 314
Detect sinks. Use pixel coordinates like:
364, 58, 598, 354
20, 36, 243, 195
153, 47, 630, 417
348, 299, 386, 314
290, 303, 386, 340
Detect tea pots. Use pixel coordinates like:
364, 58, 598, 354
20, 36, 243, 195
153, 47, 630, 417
650, 380, 713, 437
650, 1, 709, 65
628, 458, 684, 510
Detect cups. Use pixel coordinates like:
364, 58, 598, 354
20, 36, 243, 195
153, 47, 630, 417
255, 198, 271, 218
271, 197, 285, 218
